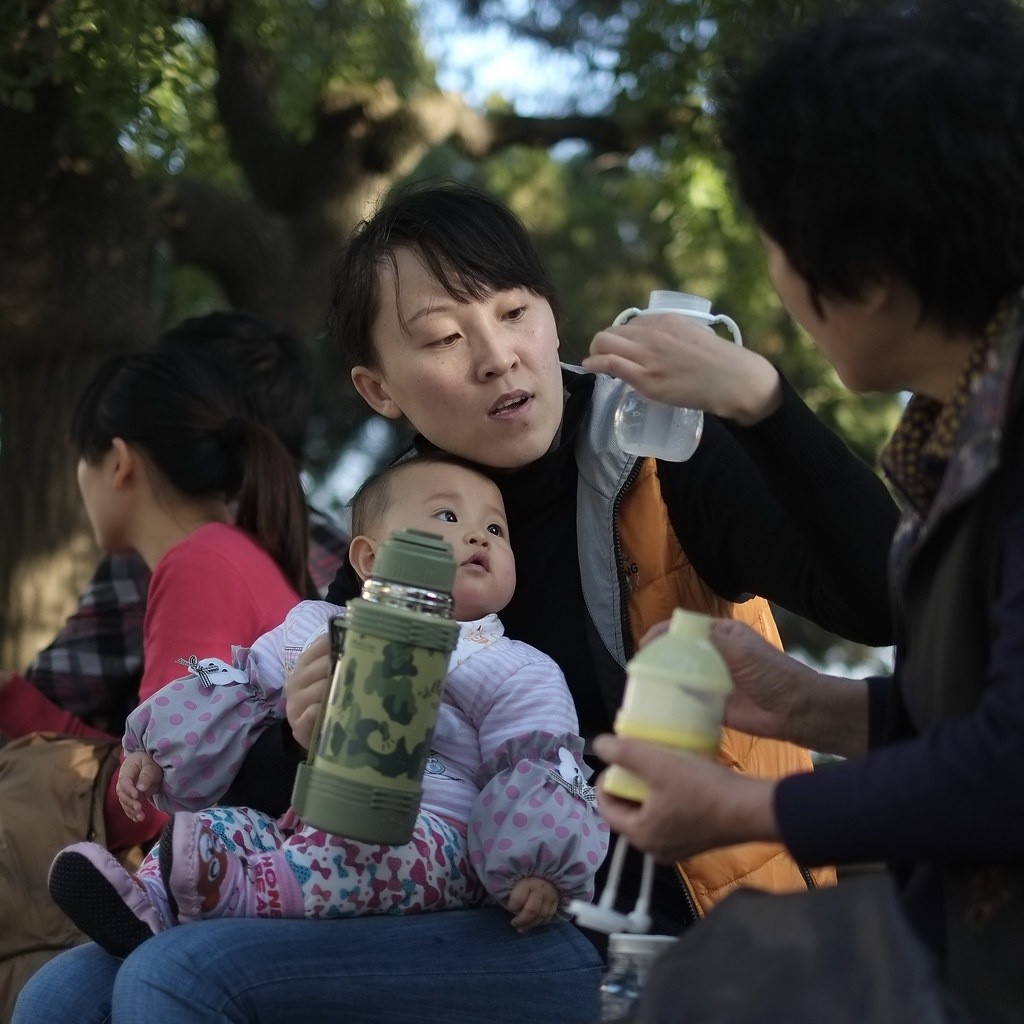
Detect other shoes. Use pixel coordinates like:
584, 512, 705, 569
159, 811, 256, 926
47, 843, 163, 962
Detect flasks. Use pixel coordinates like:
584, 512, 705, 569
293, 528, 461, 844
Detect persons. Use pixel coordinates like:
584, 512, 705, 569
11, 188, 899, 1024
46, 459, 610, 958
595, 1, 1022, 1024
0, 311, 347, 871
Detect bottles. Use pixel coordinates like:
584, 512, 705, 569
602, 608, 733, 801
599, 934, 681, 1024
609, 289, 744, 462
